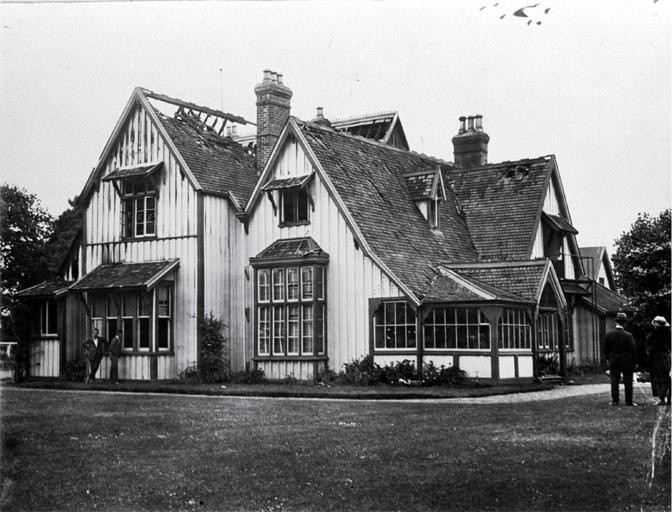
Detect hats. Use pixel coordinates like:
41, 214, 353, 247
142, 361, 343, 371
612, 312, 631, 322
651, 315, 671, 327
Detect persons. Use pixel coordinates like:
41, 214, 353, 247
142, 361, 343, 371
602, 313, 640, 406
82, 327, 110, 384
645, 315, 671, 406
108, 329, 124, 384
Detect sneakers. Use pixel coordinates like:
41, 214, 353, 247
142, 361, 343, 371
608, 400, 670, 406
